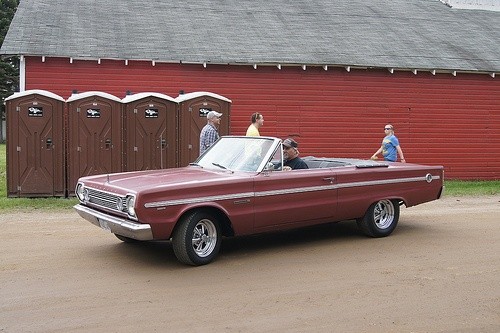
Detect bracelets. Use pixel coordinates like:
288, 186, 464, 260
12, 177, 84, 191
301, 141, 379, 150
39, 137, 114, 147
400, 159, 404, 160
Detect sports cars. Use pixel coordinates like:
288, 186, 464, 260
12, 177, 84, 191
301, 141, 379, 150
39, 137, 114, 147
71, 134, 445, 267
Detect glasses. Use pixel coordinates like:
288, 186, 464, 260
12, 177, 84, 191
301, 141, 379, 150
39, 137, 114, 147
384, 128, 391, 130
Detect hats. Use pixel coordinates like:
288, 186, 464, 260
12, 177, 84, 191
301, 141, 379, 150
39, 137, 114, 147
207, 111, 223, 120
283, 138, 299, 149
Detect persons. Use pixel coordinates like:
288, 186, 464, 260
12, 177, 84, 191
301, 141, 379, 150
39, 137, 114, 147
274, 138, 308, 169
371, 125, 406, 163
246, 112, 264, 136
200, 111, 223, 156
